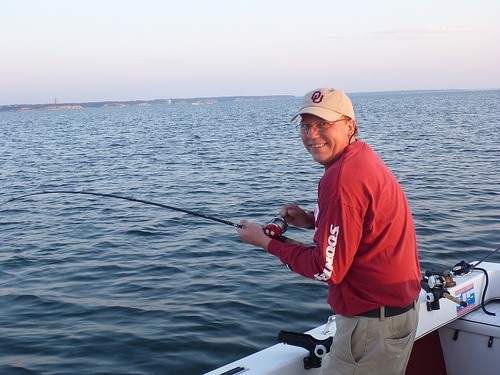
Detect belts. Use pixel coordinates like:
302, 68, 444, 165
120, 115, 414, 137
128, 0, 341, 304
355, 302, 415, 318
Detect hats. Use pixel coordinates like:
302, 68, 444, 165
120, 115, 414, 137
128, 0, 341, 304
290, 88, 355, 122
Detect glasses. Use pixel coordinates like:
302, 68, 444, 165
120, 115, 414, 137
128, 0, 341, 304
296, 119, 345, 133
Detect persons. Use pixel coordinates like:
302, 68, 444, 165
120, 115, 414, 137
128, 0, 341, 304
236, 87, 421, 375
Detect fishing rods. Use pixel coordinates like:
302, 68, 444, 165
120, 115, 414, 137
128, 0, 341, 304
0, 190, 468, 308
